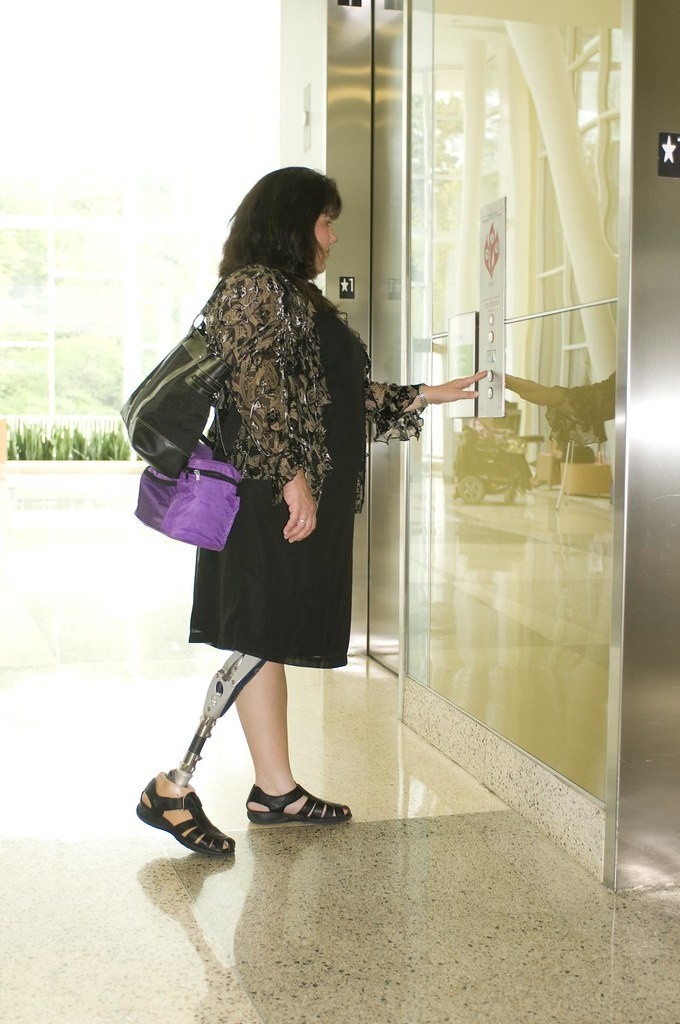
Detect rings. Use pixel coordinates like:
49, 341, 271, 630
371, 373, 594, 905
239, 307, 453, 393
298, 518, 307, 524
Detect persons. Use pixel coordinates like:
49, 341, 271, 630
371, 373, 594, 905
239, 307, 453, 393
507, 373, 614, 426
136, 165, 488, 858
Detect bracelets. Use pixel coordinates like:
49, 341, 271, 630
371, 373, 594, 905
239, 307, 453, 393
418, 386, 428, 407
553, 389, 565, 407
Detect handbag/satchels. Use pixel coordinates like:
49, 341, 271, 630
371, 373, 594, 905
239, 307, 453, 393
120, 264, 291, 478
134, 441, 243, 552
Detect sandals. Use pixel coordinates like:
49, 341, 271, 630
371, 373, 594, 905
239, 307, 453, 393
136, 778, 235, 855
246, 781, 352, 824
